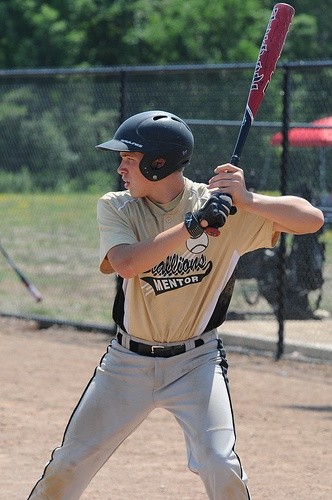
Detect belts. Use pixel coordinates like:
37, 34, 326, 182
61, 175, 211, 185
116, 331, 205, 359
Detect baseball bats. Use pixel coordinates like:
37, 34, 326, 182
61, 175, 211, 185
205, 1, 296, 237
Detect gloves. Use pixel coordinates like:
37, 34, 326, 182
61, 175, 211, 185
183, 192, 238, 239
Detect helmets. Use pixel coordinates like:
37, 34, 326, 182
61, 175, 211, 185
94, 110, 195, 181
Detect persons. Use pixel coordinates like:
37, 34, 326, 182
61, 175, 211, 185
24, 110, 326, 499
